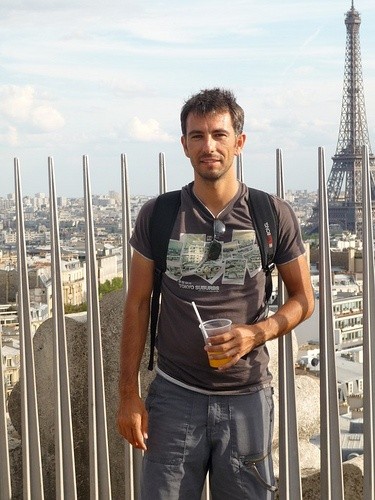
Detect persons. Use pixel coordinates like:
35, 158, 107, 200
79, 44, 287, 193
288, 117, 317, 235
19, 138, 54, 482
115, 88, 316, 500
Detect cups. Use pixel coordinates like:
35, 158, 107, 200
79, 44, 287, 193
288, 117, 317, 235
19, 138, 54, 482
198, 319, 233, 368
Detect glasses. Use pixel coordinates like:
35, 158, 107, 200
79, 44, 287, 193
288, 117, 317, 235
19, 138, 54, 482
207, 218, 227, 261
